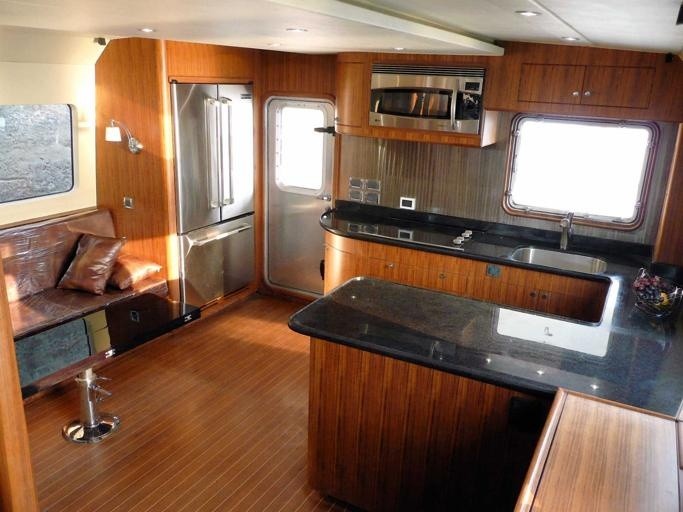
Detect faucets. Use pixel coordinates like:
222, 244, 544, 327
560, 212, 573, 251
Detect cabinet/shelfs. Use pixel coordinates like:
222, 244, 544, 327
323, 230, 611, 323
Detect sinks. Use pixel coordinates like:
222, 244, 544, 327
511, 246, 607, 275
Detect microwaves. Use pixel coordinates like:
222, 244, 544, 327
369, 64, 483, 135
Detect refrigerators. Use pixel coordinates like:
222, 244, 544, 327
173, 83, 254, 316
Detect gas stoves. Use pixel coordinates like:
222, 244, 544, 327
320, 209, 474, 251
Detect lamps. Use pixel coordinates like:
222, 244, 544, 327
105, 119, 143, 155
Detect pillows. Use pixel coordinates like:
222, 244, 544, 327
56, 233, 128, 296
107, 251, 163, 291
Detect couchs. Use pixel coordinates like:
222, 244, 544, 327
0, 206, 168, 400
